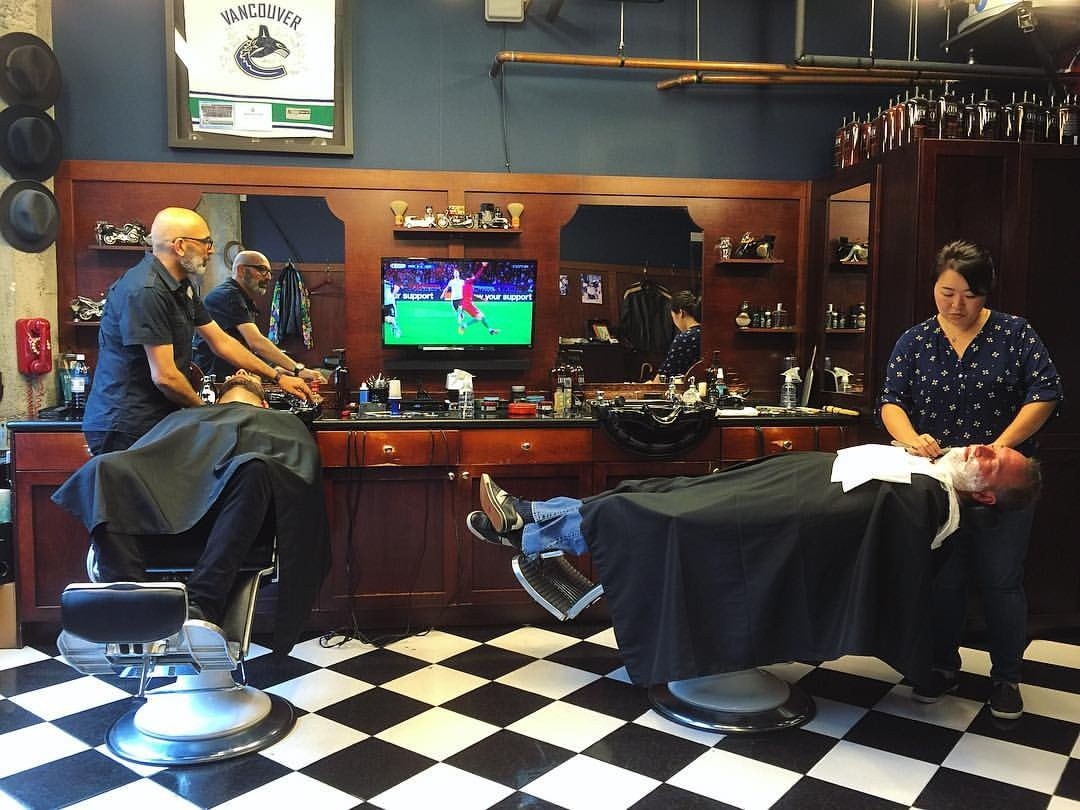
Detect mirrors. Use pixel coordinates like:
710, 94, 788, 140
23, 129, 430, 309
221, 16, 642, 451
559, 204, 704, 378
192, 192, 347, 387
825, 183, 870, 400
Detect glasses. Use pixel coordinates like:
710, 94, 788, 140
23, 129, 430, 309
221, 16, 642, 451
173, 236, 214, 249
243, 264, 272, 280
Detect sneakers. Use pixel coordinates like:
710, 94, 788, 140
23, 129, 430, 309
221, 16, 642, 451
186, 599, 225, 630
458, 312, 464, 322
457, 324, 466, 333
466, 511, 519, 547
911, 671, 963, 704
392, 326, 400, 337
988, 679, 1024, 719
480, 473, 523, 533
488, 328, 502, 335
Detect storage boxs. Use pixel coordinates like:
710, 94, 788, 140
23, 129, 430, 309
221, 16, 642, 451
0, 581, 18, 649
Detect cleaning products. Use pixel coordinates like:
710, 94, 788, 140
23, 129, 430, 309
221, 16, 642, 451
834, 366, 855, 393
452, 367, 477, 418
779, 366, 803, 408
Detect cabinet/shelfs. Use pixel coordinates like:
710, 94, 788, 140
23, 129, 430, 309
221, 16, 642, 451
827, 256, 866, 336
312, 427, 591, 616
590, 423, 857, 623
716, 253, 800, 336
812, 137, 1080, 636
9, 432, 279, 626
66, 242, 154, 332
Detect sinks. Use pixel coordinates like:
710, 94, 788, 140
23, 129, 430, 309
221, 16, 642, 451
587, 397, 718, 464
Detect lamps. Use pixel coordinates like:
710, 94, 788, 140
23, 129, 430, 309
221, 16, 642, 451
940, 0, 1080, 105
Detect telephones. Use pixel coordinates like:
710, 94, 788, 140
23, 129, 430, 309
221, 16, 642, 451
16, 318, 53, 377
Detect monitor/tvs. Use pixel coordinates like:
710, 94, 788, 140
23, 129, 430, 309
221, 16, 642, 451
379, 256, 535, 351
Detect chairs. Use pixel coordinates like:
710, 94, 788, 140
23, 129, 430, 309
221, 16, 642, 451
55, 530, 297, 770
469, 511, 820, 734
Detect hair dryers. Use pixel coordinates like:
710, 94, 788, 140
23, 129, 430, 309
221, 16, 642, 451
323, 355, 349, 403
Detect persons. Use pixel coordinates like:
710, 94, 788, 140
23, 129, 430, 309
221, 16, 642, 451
191, 250, 328, 386
382, 273, 400, 339
872, 238, 1066, 721
81, 206, 318, 460
440, 268, 465, 324
382, 259, 534, 291
458, 261, 501, 336
464, 442, 1043, 560
652, 290, 703, 384
89, 366, 322, 631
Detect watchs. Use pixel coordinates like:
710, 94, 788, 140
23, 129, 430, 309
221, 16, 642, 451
294, 362, 305, 378
271, 368, 284, 386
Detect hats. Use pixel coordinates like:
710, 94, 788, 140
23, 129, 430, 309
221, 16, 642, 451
1, 179, 60, 252
0, 32, 62, 113
0, 107, 62, 180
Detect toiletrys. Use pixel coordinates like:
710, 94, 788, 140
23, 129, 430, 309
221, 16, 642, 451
717, 229, 778, 261
359, 366, 403, 419
702, 348, 752, 412
550, 362, 592, 419
734, 298, 787, 328
825, 300, 868, 330
831, 83, 1080, 180
196, 374, 217, 403
682, 377, 704, 408
835, 235, 868, 263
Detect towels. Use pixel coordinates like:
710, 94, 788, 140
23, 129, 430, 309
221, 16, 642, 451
831, 441, 946, 498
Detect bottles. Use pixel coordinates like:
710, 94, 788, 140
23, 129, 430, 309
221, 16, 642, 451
553, 385, 565, 414
830, 63, 1080, 169
57, 352, 79, 402
737, 301, 790, 331
666, 368, 728, 406
512, 385, 528, 403
71, 354, 91, 411
779, 367, 801, 408
360, 382, 369, 404
822, 298, 867, 331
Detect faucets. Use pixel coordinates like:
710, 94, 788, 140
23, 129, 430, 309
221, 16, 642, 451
596, 390, 606, 402
663, 375, 684, 402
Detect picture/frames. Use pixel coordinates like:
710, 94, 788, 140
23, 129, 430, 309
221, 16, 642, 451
164, 0, 354, 159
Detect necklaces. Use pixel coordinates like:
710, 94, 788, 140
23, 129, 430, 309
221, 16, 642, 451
939, 308, 989, 343
